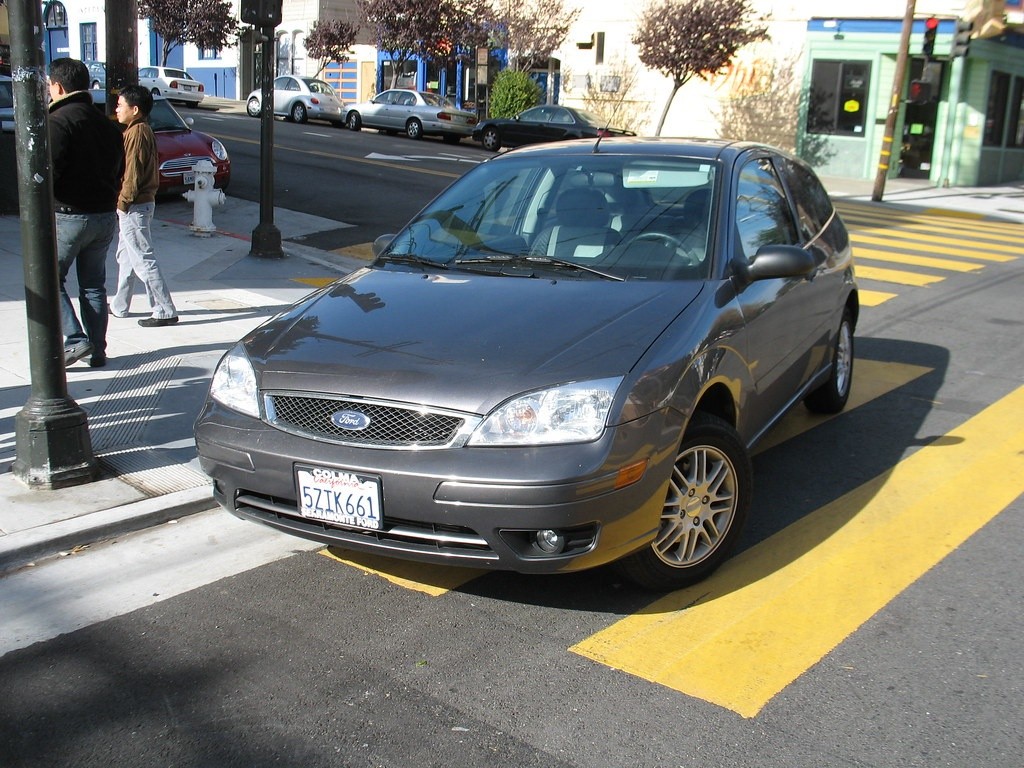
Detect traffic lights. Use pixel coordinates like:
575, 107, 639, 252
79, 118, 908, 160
924, 18, 937, 52
910, 80, 920, 99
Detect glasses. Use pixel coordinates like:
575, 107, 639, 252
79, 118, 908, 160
117, 104, 136, 110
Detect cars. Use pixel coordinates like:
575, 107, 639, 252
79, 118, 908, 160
472, 105, 637, 151
194, 136, 860, 590
82, 60, 106, 89
137, 66, 204, 109
0, 46, 11, 76
0, 75, 15, 131
84, 89, 231, 195
246, 75, 347, 128
343, 89, 478, 144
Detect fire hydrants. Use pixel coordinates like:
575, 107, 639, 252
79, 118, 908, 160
183, 160, 226, 236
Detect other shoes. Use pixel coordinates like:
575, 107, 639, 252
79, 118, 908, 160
107, 303, 112, 314
80, 350, 106, 367
63, 332, 94, 367
138, 317, 178, 327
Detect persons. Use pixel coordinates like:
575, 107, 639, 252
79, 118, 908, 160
47, 56, 127, 368
107, 86, 179, 327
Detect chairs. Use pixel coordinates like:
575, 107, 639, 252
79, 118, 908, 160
662, 188, 712, 262
527, 188, 622, 264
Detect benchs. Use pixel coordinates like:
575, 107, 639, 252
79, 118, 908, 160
600, 189, 684, 243
